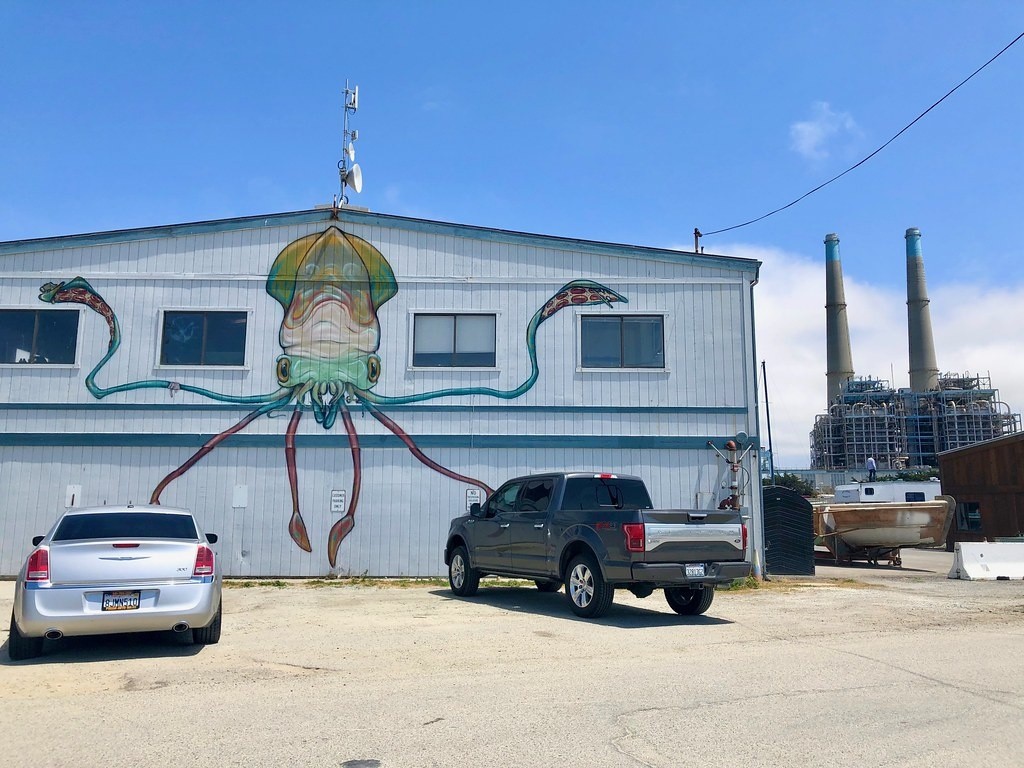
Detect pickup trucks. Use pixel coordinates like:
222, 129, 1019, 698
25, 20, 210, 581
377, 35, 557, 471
444, 470, 750, 619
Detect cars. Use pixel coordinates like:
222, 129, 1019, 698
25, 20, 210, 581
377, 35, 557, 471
9, 504, 222, 660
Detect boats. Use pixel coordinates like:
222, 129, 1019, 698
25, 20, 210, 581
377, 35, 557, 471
812, 494, 957, 557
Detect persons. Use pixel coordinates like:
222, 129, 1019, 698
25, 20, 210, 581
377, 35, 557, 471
867, 457, 877, 482
